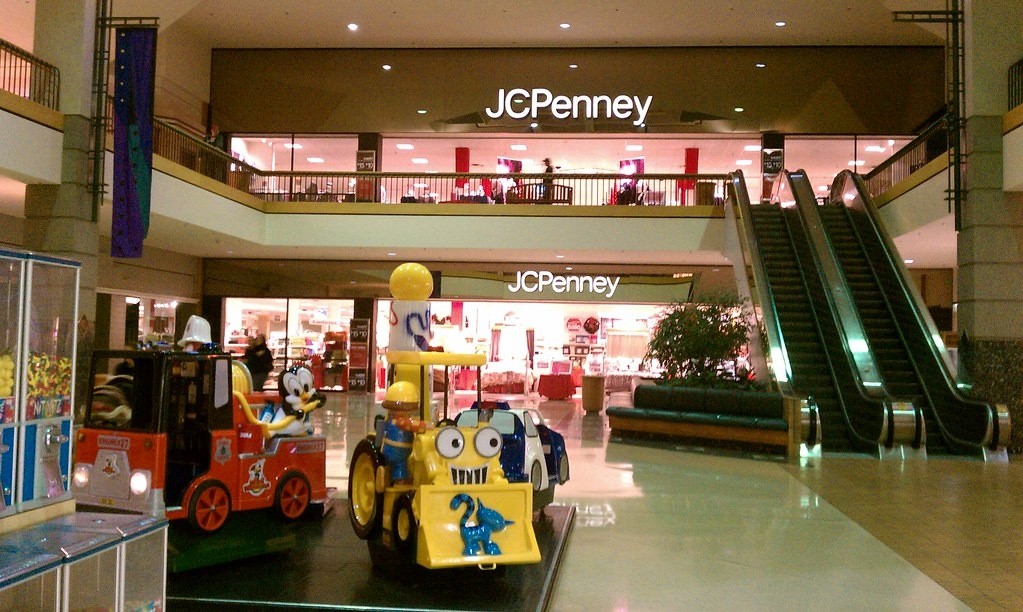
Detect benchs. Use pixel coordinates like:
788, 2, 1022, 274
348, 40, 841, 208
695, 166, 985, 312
505, 183, 572, 205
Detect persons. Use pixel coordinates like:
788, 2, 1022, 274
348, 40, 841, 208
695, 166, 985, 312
543, 157, 552, 204
379, 380, 434, 488
238, 334, 274, 392
